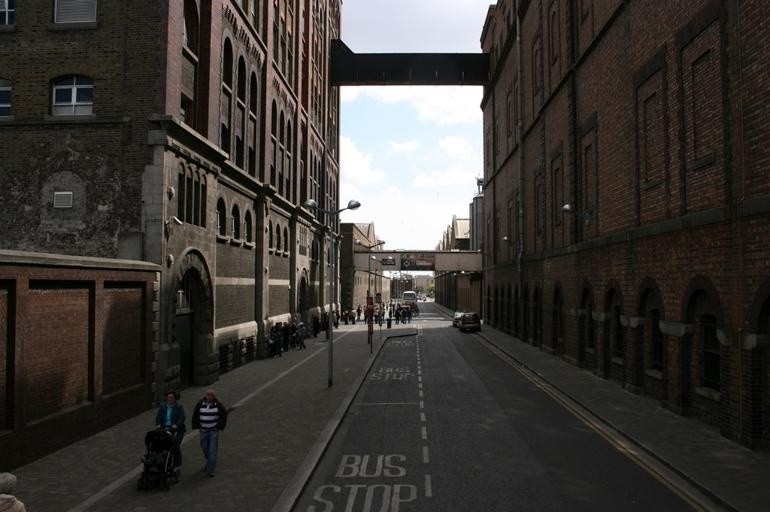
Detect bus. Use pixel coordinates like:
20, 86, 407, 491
402, 291, 418, 305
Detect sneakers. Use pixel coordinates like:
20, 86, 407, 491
202, 465, 208, 471
207, 471, 215, 477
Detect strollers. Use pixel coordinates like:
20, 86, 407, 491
137, 425, 181, 490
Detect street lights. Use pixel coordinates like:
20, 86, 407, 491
355, 236, 393, 297
304, 199, 360, 388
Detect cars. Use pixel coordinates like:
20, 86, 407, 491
453, 312, 481, 331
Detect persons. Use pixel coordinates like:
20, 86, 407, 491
0, 472, 27, 512
265, 297, 420, 364
192, 388, 228, 478
139, 438, 167, 468
154, 390, 185, 485
